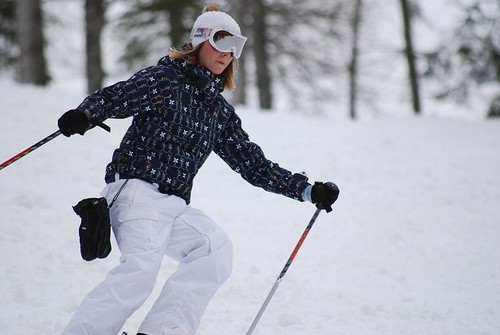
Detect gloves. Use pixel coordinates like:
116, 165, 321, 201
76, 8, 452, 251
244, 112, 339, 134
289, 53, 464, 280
310, 181, 338, 210
73, 197, 111, 260
58, 110, 87, 137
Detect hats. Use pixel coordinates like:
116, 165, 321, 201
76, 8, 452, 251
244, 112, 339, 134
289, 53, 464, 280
191, 10, 241, 52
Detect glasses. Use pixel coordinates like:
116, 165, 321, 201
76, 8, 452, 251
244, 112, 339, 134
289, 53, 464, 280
196, 27, 247, 58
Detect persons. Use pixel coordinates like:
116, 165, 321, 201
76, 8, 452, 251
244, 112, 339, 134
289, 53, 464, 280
57, 6, 340, 335
